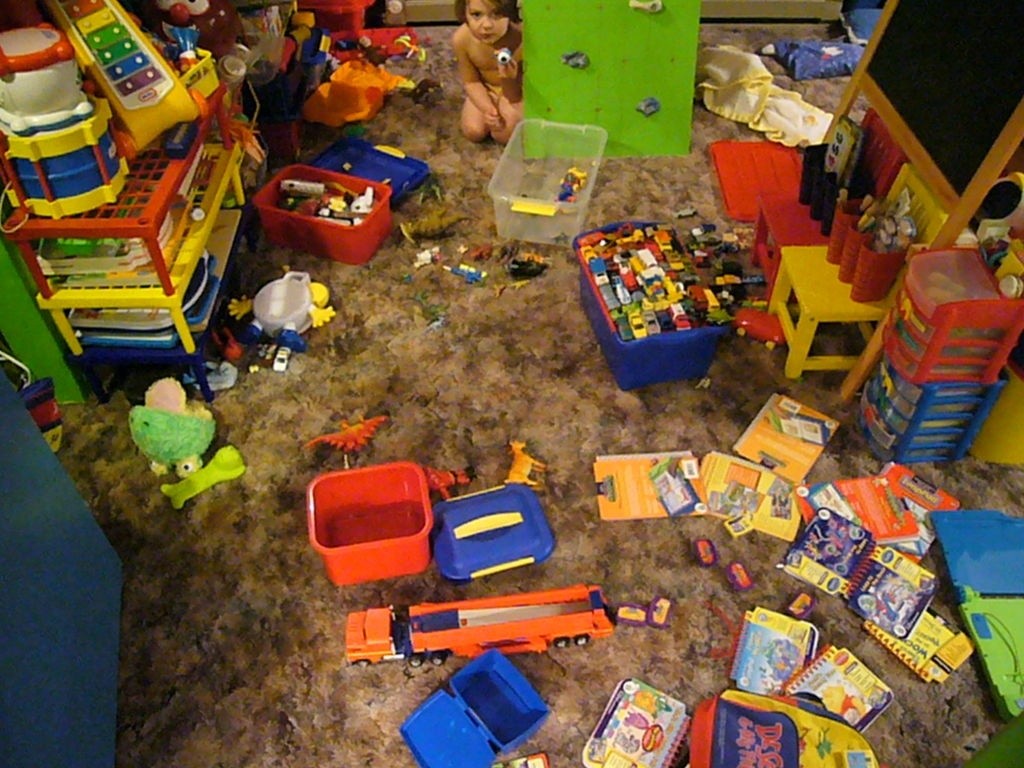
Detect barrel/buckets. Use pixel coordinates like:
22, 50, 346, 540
253, 272, 312, 335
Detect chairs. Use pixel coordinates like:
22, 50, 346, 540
752, 107, 911, 296
768, 163, 950, 375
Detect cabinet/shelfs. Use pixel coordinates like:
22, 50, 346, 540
4, 143, 257, 402
860, 242, 1024, 464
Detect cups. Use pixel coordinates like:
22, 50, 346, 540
798, 142, 907, 303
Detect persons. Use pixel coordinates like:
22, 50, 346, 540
451, 0, 523, 144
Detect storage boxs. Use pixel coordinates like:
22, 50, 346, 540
307, 461, 433, 584
253, 163, 394, 266
178, 47, 221, 98
399, 648, 551, 768
488, 120, 608, 245
573, 221, 728, 389
